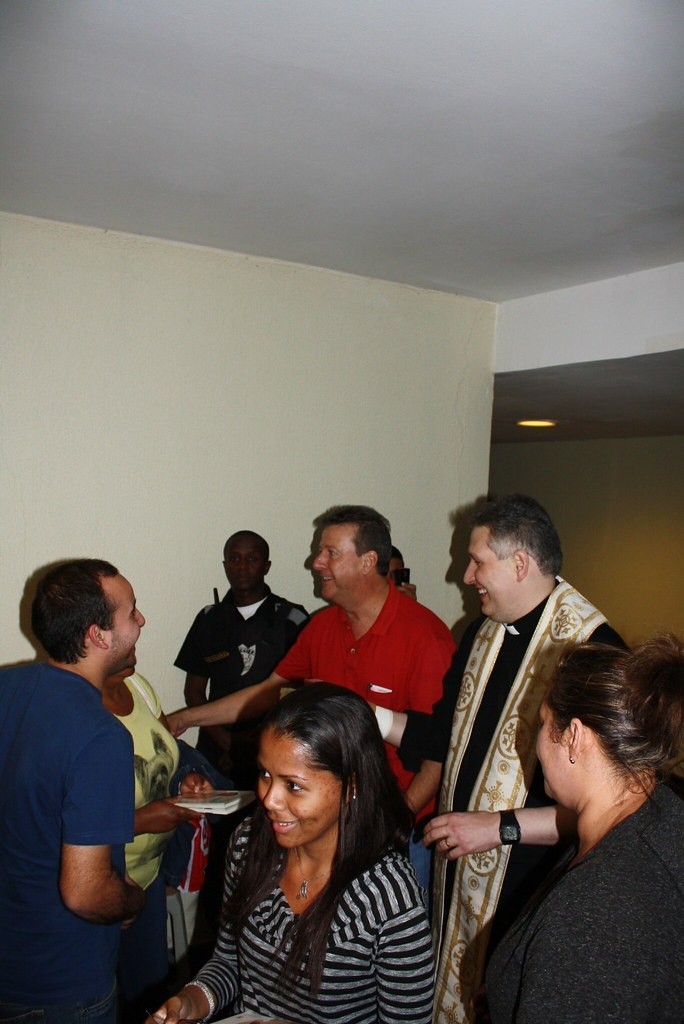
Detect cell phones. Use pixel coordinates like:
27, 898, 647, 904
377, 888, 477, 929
395, 568, 411, 587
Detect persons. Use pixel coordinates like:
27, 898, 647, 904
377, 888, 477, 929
144, 682, 433, 1024
468, 634, 684, 1024
171, 527, 314, 785
388, 547, 417, 599
165, 505, 458, 920
282, 496, 633, 1024
0, 557, 206, 1024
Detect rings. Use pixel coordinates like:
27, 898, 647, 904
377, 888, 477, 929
445, 840, 451, 848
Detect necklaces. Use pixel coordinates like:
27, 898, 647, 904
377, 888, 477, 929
292, 849, 331, 898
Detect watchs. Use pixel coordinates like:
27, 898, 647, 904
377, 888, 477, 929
499, 808, 520, 844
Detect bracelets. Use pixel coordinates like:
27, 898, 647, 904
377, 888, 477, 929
185, 980, 215, 1022
